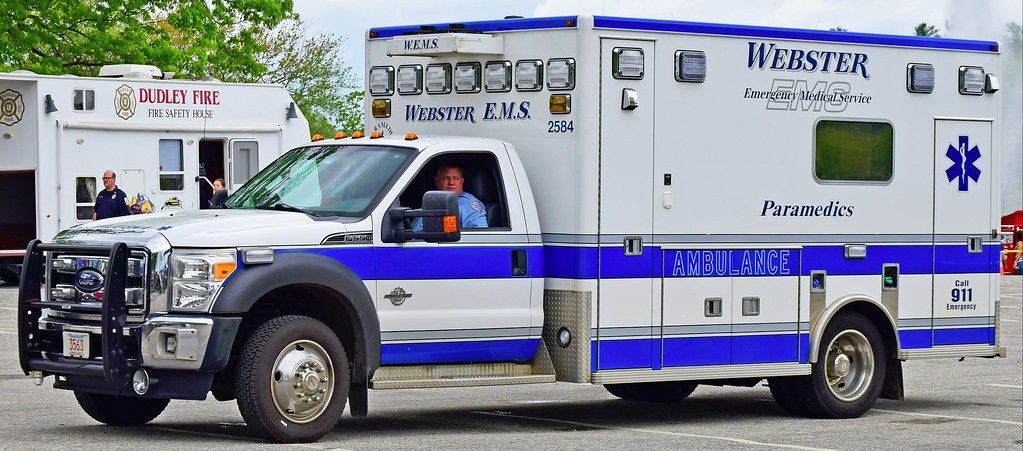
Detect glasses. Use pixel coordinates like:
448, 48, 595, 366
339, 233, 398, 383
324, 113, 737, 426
102, 177, 115, 181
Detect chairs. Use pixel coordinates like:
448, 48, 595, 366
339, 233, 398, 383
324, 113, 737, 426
463, 165, 501, 227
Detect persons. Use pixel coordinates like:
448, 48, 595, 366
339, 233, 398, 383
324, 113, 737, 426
410, 165, 488, 233
208, 178, 225, 209
93, 170, 132, 219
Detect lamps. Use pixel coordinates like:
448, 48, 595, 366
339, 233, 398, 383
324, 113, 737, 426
286, 102, 298, 119
45, 93, 59, 114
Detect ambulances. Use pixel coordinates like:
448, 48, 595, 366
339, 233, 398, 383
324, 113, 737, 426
16, 17, 1014, 445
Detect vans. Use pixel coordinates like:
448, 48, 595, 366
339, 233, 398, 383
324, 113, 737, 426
1, 65, 322, 286
